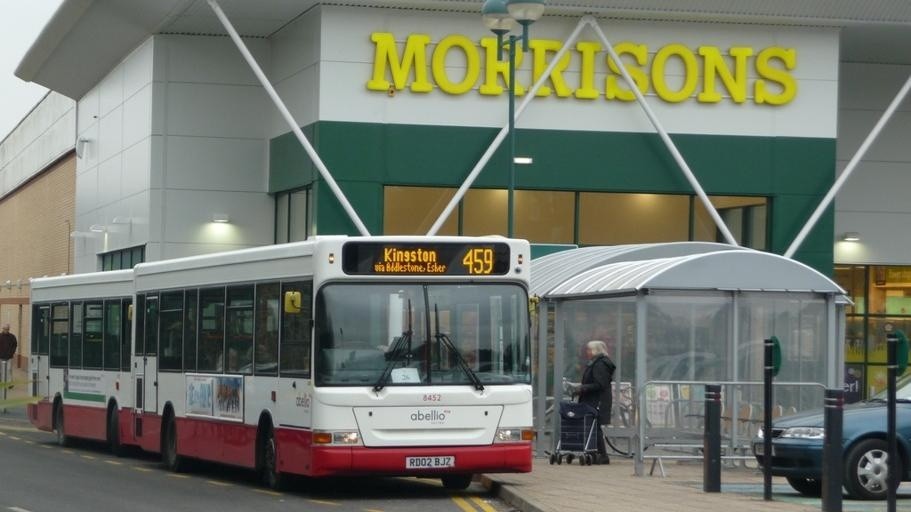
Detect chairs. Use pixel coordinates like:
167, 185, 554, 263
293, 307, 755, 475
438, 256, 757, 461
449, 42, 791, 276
44, 332, 403, 372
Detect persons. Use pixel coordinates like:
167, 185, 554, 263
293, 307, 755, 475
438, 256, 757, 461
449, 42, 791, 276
1, 323, 18, 390
163, 308, 396, 382
572, 340, 618, 465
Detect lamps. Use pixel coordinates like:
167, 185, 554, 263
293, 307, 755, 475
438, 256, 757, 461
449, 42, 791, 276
513, 157, 533, 164
90, 225, 105, 232
76, 136, 88, 159
113, 216, 131, 224
842, 232, 861, 240
214, 213, 228, 222
71, 231, 84, 237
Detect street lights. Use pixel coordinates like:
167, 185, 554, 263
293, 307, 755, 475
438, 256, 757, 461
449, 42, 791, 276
480, 2, 546, 237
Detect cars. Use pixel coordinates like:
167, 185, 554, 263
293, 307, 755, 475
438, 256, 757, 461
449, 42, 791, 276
749, 367, 911, 501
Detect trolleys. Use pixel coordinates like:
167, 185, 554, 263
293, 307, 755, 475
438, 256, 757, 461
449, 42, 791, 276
549, 390, 603, 466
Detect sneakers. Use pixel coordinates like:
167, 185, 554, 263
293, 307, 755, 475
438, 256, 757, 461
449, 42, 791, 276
593, 454, 610, 464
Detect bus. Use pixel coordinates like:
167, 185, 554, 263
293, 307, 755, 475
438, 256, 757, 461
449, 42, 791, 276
129, 232, 535, 491
27, 269, 146, 459
